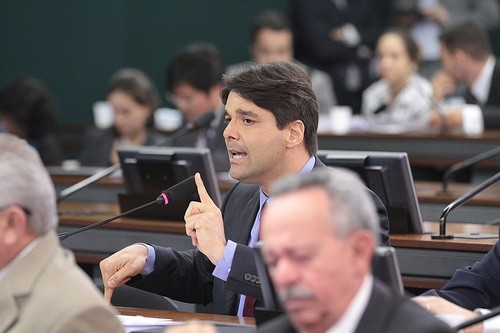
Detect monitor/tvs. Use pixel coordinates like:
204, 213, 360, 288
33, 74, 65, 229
116, 145, 223, 222
315, 149, 425, 235
251, 242, 405, 311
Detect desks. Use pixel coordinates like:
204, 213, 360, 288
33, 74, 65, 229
47, 130, 500, 333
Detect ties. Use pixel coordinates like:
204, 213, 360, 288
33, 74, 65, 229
242, 201, 269, 317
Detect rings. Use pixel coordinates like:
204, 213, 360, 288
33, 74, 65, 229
426, 304, 431, 312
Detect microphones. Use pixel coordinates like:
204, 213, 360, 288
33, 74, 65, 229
154, 111, 215, 147
56, 175, 199, 241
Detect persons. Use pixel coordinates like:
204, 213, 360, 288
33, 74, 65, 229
0, 132, 125, 332
100, 62, 389, 317
412, 231, 500, 333
224, 14, 335, 113
293, 0, 500, 133
0, 79, 49, 139
89, 71, 166, 166
257, 168, 453, 333
166, 48, 231, 173
